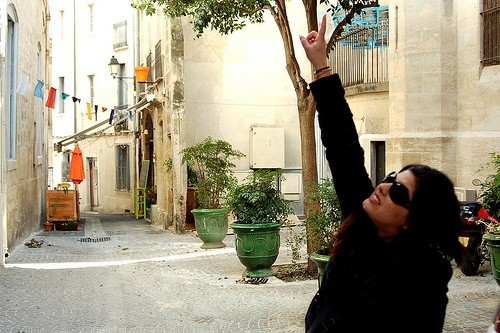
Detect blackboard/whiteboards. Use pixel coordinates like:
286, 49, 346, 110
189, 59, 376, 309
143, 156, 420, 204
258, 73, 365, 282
138, 160, 151, 189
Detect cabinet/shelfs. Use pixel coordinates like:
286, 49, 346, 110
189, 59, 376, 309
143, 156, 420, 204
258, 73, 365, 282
46, 190, 81, 222
134, 188, 146, 219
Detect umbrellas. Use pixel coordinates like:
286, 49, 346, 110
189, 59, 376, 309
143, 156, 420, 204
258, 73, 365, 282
70, 146, 85, 190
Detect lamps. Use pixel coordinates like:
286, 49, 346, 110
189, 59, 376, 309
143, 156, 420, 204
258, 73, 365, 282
108, 56, 133, 80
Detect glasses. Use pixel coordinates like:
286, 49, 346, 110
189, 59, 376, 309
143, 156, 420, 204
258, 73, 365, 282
381, 170, 414, 212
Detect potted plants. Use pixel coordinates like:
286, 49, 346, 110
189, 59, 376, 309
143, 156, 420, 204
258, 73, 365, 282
222, 168, 295, 278
302, 176, 342, 291
477, 152, 500, 286
178, 136, 247, 249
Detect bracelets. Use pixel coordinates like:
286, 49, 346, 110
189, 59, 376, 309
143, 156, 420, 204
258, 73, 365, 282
314, 66, 330, 74
315, 69, 332, 78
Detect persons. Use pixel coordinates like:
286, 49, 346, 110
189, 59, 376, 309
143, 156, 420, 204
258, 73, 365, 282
299, 15, 460, 333
